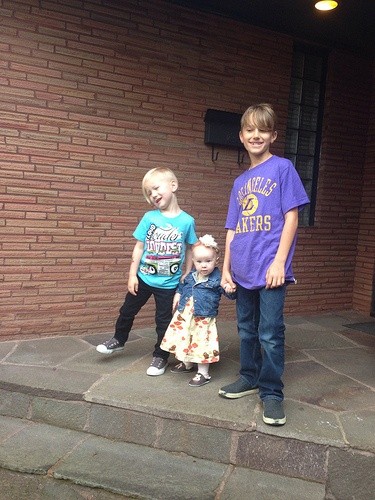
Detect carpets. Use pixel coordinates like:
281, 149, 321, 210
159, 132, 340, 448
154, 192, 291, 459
343, 322, 375, 336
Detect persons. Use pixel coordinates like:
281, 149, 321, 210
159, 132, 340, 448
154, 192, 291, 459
220, 103, 310, 426
159, 233, 238, 386
96, 166, 198, 376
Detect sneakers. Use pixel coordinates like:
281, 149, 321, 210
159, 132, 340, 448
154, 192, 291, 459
170, 363, 194, 373
96, 337, 124, 354
263, 399, 286, 424
189, 374, 211, 387
219, 378, 259, 398
147, 357, 169, 375
